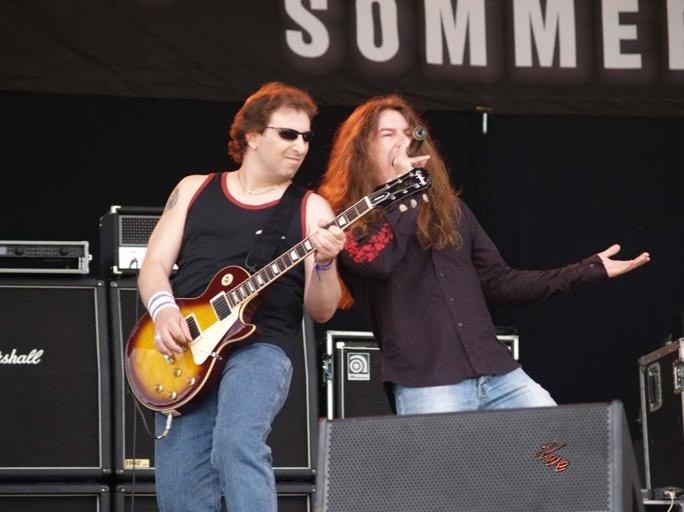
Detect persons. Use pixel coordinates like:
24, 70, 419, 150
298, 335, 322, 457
137, 79, 348, 511
318, 94, 653, 416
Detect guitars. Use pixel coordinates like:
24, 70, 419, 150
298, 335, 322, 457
123, 166, 432, 415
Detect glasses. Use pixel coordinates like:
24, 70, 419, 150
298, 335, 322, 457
265, 125, 315, 142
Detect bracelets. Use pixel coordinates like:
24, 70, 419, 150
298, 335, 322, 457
147, 290, 179, 323
312, 259, 335, 283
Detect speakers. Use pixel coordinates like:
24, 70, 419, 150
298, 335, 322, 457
312, 399, 645, 512
0, 284, 112, 480
109, 281, 316, 481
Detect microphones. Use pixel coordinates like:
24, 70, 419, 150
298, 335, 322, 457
406, 127, 425, 157
